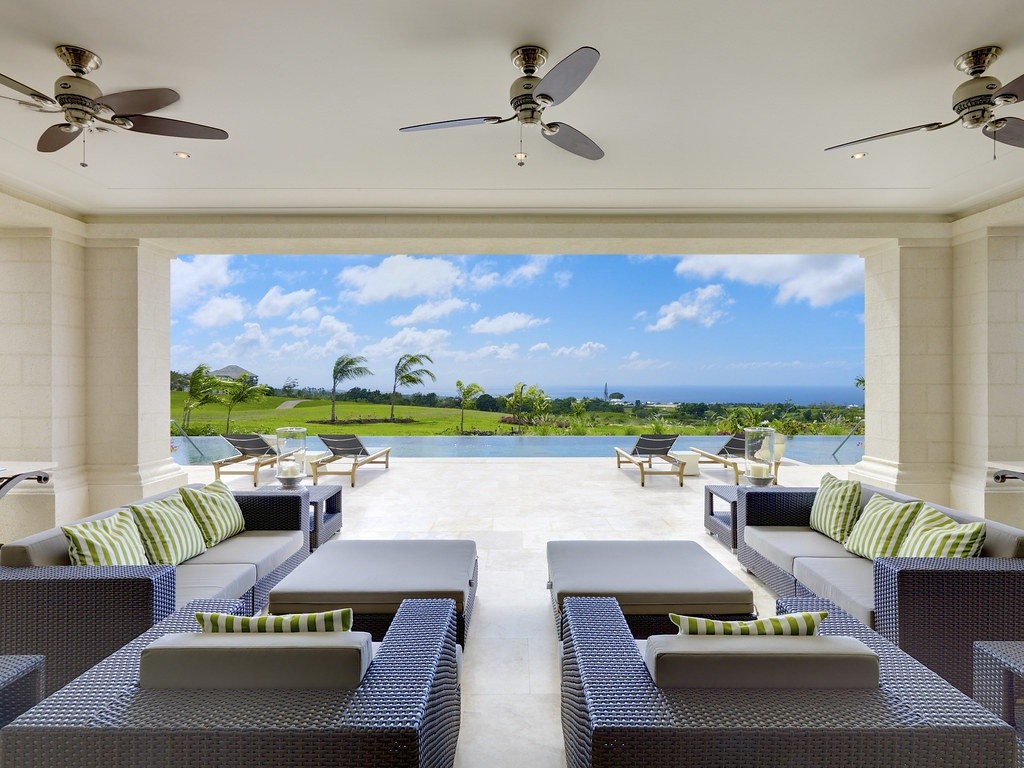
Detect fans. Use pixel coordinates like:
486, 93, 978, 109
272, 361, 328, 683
0, 47, 225, 167
400, 46, 605, 165
823, 47, 1024, 152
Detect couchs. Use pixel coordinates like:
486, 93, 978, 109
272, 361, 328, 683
562, 594, 1018, 768
0, 480, 309, 696
737, 479, 1024, 698
0, 598, 462, 768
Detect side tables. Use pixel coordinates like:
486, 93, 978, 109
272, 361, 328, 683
972, 641, 1024, 768
264, 485, 345, 549
704, 484, 784, 553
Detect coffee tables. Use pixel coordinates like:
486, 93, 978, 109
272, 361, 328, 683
267, 540, 478, 654
547, 535, 756, 643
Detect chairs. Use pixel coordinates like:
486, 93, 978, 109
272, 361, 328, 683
310, 433, 392, 488
213, 431, 306, 487
689, 427, 779, 486
614, 432, 681, 486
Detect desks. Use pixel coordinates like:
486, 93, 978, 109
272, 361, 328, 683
669, 452, 701, 477
292, 451, 328, 476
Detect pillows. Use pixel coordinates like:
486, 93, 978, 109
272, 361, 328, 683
195, 606, 353, 634
130, 494, 208, 568
182, 478, 247, 538
58, 508, 145, 570
668, 610, 828, 635
844, 491, 921, 558
190, 608, 354, 637
805, 468, 863, 541
898, 506, 984, 564
